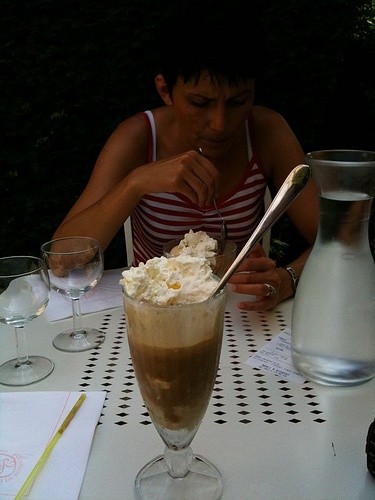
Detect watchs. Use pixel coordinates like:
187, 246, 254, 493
285, 266, 299, 292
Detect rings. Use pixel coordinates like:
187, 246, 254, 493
264, 283, 276, 298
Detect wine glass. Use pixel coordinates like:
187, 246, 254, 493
39, 235, 106, 352
0, 255, 55, 387
121, 272, 224, 500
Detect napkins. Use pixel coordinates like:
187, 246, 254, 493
26, 265, 130, 322
0, 392, 107, 499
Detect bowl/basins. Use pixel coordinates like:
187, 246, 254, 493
163, 235, 238, 278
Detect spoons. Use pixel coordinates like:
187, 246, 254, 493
199, 148, 228, 255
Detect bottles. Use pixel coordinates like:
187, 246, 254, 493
290, 149, 375, 386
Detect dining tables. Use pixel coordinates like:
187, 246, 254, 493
0, 266, 375, 500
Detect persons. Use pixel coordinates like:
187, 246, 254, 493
50, 10, 321, 313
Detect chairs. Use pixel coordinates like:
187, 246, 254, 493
123, 184, 271, 265
